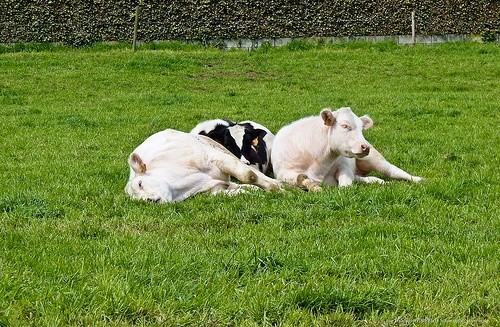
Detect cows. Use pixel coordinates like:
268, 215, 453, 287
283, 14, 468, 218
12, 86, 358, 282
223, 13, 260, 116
188, 119, 274, 176
270, 104, 422, 193
124, 128, 281, 206
